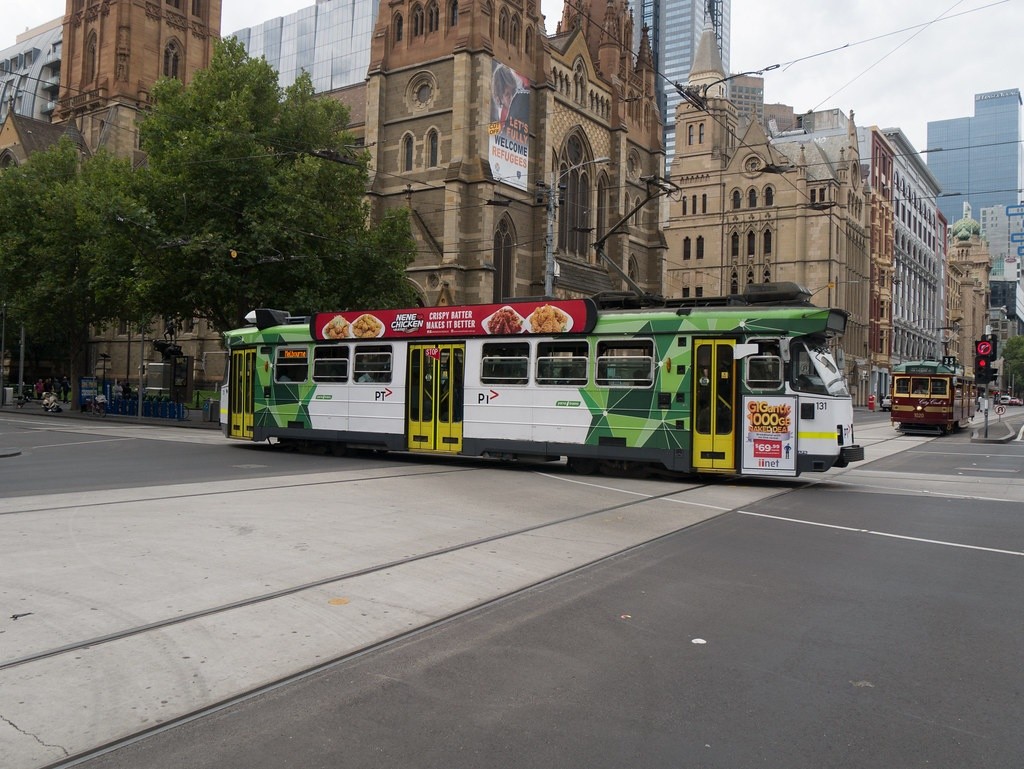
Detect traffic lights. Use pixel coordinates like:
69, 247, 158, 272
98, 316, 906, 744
975, 341, 991, 384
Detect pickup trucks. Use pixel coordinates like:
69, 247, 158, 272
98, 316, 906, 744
882, 395, 892, 410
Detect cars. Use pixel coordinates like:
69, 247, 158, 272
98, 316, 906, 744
999, 395, 1023, 405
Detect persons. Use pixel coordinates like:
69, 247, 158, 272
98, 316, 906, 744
633, 370, 642, 386
36, 376, 71, 404
491, 65, 518, 130
540, 367, 555, 385
163, 321, 176, 342
752, 358, 772, 386
557, 367, 569, 384
113, 381, 132, 398
913, 384, 927, 393
359, 364, 375, 382
976, 395, 983, 412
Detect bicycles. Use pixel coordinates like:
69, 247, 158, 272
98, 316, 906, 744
157, 399, 190, 419
92, 398, 106, 417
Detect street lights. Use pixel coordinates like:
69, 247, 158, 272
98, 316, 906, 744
546, 157, 611, 297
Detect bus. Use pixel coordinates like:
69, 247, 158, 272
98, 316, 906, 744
218, 282, 864, 480
890, 358, 978, 434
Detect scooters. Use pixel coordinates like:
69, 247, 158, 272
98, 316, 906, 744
42, 391, 59, 411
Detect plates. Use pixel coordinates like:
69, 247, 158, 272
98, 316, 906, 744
349, 314, 385, 337
481, 306, 527, 334
322, 316, 352, 339
524, 305, 574, 333
